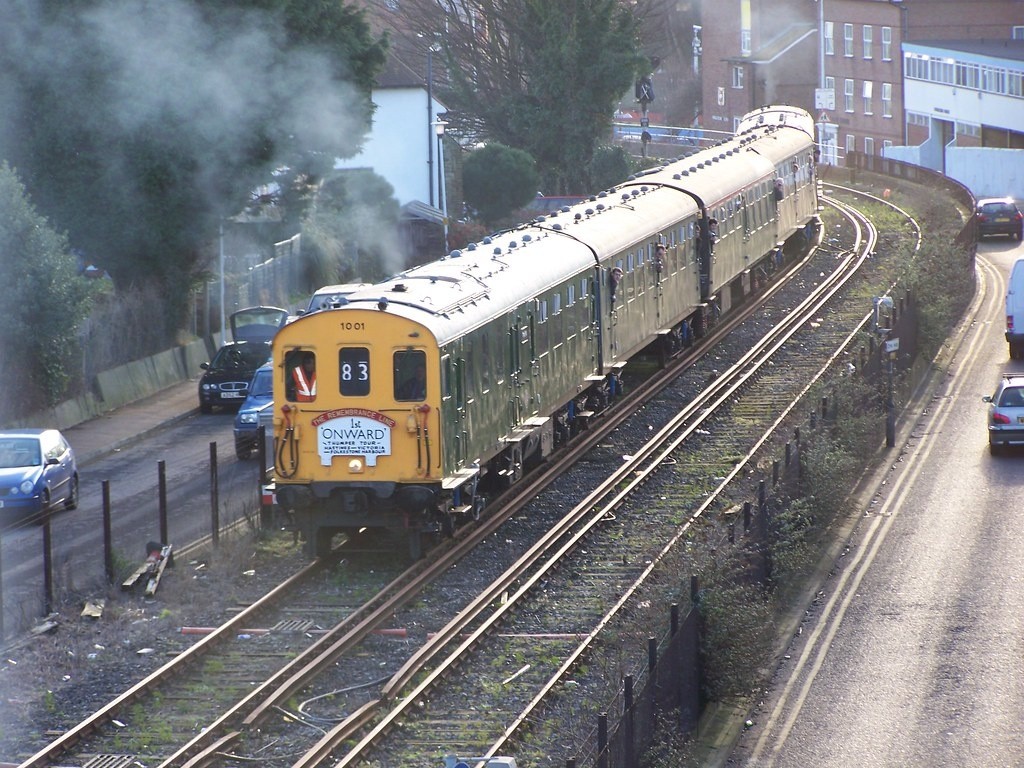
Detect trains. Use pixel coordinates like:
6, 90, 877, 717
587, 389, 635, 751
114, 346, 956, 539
270, 103, 822, 560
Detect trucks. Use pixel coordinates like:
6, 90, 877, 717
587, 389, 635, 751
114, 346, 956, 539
1004, 253, 1024, 360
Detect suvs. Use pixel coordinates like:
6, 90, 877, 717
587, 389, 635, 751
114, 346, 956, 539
199, 306, 290, 415
233, 359, 272, 459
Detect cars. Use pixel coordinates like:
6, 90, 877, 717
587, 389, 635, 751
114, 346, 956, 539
296, 283, 373, 319
982, 373, 1024, 455
976, 196, 1024, 241
0, 428, 80, 525
